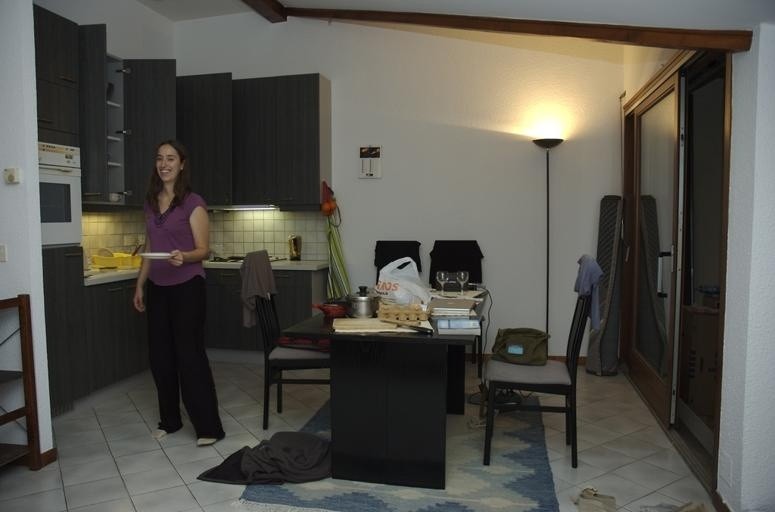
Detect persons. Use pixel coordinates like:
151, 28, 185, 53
132, 139, 225, 447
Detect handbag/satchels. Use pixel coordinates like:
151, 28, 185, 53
490, 327, 551, 367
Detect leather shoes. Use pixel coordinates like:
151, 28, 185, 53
568, 486, 617, 511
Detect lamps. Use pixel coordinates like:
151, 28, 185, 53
532, 139, 564, 359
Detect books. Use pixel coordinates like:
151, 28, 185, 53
429, 298, 482, 336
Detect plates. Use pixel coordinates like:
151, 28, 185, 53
137, 252, 177, 260
98, 247, 114, 257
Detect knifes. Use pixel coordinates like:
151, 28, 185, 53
379, 320, 434, 334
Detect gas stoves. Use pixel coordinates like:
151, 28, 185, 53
209, 253, 287, 264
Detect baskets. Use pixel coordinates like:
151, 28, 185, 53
92, 252, 142, 269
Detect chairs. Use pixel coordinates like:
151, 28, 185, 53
483, 255, 596, 468
375, 240, 484, 379
246, 250, 330, 430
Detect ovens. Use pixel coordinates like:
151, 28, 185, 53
38, 142, 84, 250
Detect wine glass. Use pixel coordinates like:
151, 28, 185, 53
455, 271, 469, 296
436, 271, 450, 296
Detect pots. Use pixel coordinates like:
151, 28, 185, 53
309, 297, 347, 317
343, 285, 383, 318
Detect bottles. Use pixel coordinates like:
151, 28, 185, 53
287, 233, 299, 261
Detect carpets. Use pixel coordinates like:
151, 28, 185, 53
231, 391, 560, 512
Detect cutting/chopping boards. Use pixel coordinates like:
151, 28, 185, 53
332, 316, 435, 334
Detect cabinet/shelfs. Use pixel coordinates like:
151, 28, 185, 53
0, 294, 42, 471
42, 246, 147, 419
202, 267, 328, 352
